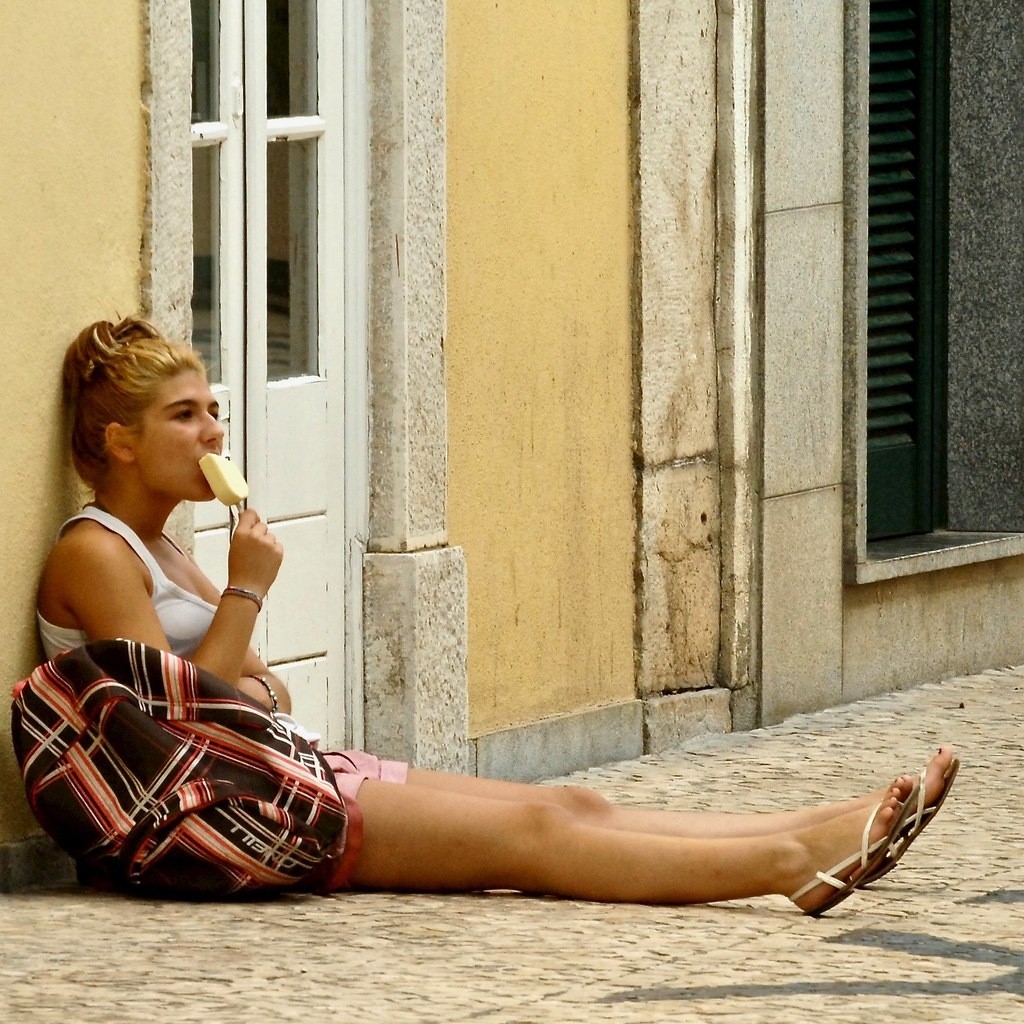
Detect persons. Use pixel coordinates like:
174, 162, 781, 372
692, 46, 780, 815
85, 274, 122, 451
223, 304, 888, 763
10, 316, 959, 914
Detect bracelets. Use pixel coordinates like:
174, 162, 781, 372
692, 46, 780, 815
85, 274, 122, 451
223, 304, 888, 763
220, 587, 263, 612
247, 672, 278, 713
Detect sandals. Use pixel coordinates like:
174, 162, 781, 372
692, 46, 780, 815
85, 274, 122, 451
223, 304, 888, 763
855, 758, 960, 887
789, 774, 921, 916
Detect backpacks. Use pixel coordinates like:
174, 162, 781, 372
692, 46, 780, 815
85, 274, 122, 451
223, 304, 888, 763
10, 638, 348, 896
